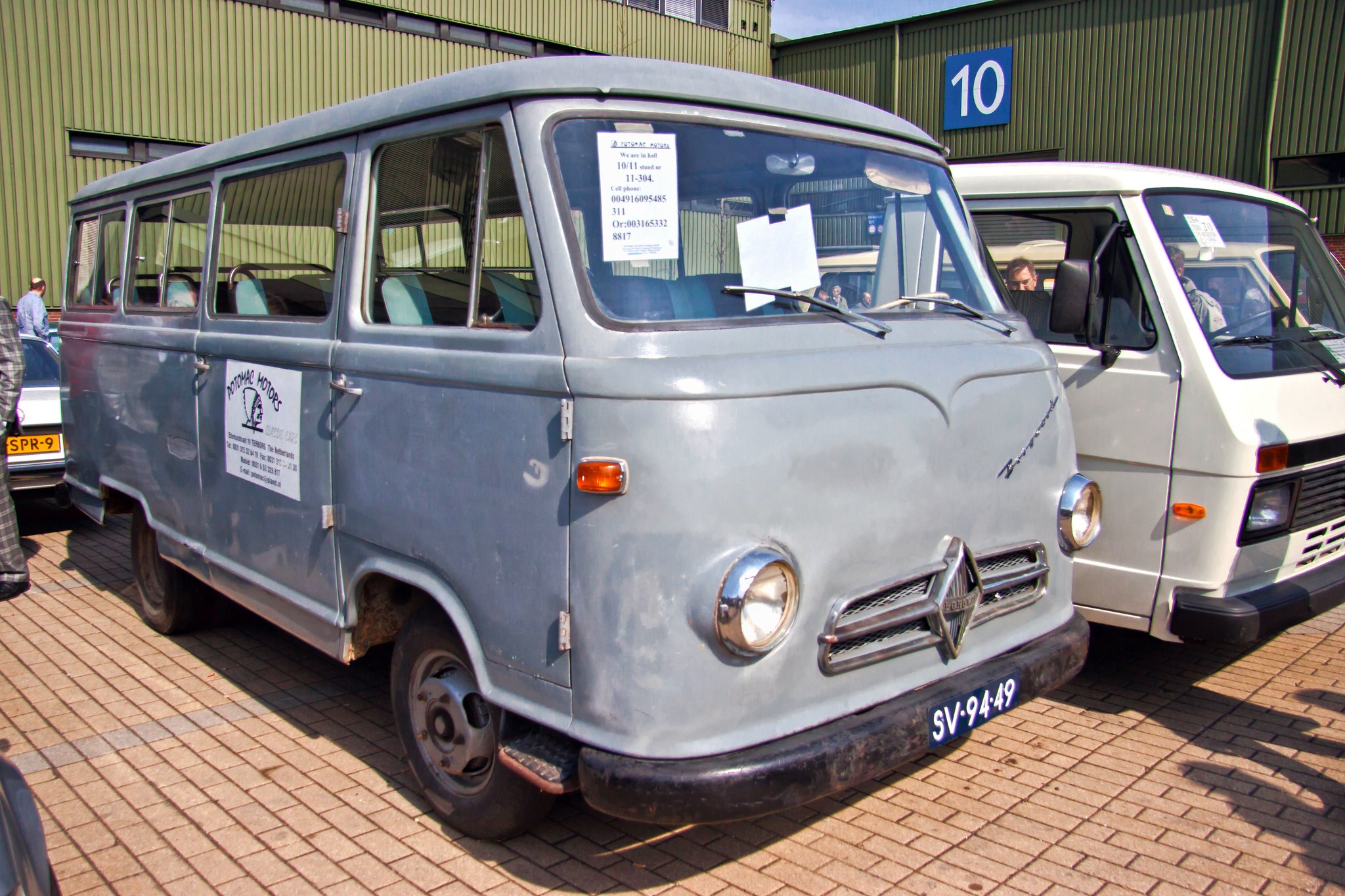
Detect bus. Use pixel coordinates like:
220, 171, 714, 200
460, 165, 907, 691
62, 55, 1105, 845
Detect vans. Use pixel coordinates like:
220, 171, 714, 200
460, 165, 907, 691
793, 240, 1344, 335
945, 162, 1345, 652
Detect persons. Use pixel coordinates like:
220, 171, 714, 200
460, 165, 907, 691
1005, 256, 1038, 291
0, 296, 31, 602
818, 290, 829, 302
1242, 286, 1271, 329
17, 278, 53, 345
826, 286, 848, 309
856, 292, 871, 309
1144, 244, 1230, 336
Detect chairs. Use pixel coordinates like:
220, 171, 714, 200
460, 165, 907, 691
1008, 289, 1077, 344
1089, 296, 1150, 350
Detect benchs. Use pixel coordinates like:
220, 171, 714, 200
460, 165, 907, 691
106, 263, 804, 328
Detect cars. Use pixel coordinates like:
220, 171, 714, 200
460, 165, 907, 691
5, 332, 73, 511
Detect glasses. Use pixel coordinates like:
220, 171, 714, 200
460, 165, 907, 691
1008, 279, 1034, 288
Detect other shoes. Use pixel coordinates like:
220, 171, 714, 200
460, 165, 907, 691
0, 582, 32, 600
